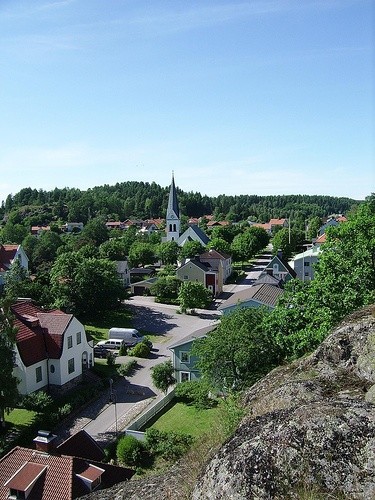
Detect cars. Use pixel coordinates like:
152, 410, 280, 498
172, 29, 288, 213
94, 346, 112, 358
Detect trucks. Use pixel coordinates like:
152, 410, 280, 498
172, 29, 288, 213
108, 327, 146, 346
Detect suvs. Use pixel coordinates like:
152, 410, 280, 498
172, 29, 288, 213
98, 339, 125, 349
